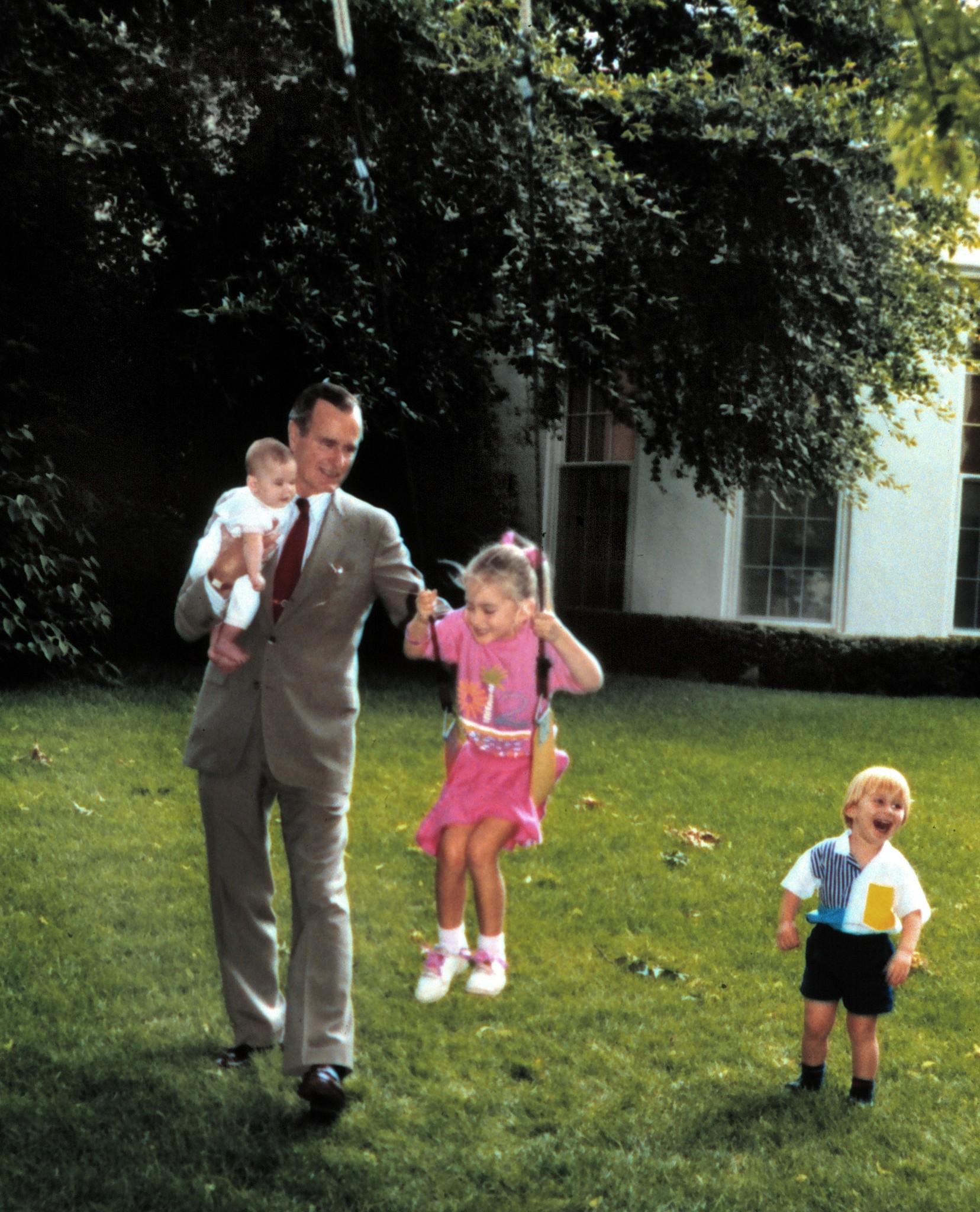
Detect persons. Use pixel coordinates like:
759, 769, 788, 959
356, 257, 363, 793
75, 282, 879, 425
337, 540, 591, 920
776, 764, 933, 1109
188, 438, 299, 671
175, 382, 447, 1124
403, 531, 602, 1005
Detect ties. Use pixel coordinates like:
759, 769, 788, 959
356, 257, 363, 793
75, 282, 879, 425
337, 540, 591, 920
273, 497, 310, 629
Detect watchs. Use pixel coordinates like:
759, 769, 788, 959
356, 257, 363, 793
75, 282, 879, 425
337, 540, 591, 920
207, 570, 231, 591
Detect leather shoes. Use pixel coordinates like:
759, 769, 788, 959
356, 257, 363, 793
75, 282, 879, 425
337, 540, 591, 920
297, 1065, 346, 1124
213, 1044, 284, 1069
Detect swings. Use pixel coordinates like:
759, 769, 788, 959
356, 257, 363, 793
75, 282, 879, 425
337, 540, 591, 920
332, 0, 555, 805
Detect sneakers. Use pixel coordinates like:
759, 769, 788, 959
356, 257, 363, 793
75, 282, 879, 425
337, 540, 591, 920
458, 944, 510, 995
414, 933, 470, 1002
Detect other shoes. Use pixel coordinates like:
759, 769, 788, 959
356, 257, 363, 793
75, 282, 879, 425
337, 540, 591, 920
842, 1095, 875, 1114
784, 1082, 822, 1094
